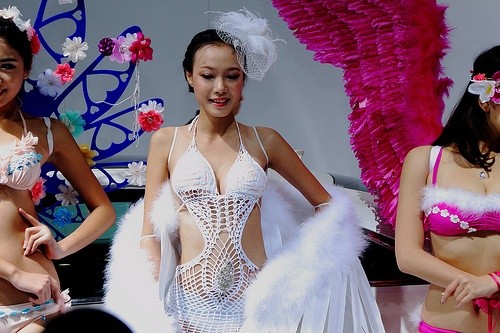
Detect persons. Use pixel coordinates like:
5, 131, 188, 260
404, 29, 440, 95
395, 44, 500, 333
103, 7, 385, 333
0, 6, 116, 333
40, 306, 134, 333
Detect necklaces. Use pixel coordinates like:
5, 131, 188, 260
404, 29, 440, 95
480, 153, 500, 179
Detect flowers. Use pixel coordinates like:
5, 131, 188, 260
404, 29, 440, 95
63, 36, 88, 62
467, 71, 500, 105
125, 161, 147, 187
137, 101, 166, 132
52, 182, 78, 228
0, 6, 40, 56
59, 109, 85, 140
54, 65, 75, 84
98, 32, 153, 64
37, 70, 62, 96
79, 145, 98, 167
